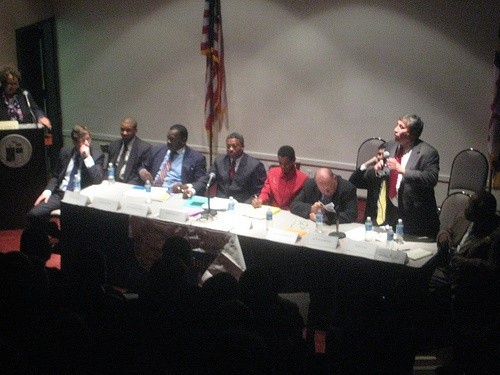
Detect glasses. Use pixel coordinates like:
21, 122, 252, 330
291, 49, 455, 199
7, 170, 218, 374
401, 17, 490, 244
226, 143, 240, 148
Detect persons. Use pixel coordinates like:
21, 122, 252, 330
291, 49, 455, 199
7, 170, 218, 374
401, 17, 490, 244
374, 114, 440, 239
0, 64, 52, 130
183, 132, 267, 204
350, 143, 398, 233
427, 190, 500, 300
138, 125, 207, 193
0, 226, 426, 375
25, 125, 104, 235
105, 117, 153, 186
290, 168, 358, 225
252, 145, 310, 211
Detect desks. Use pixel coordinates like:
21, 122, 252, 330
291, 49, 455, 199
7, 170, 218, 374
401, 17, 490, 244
59, 178, 439, 320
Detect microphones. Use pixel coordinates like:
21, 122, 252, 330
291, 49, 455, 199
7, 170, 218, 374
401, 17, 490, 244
360, 151, 390, 171
207, 173, 216, 187
23, 90, 32, 108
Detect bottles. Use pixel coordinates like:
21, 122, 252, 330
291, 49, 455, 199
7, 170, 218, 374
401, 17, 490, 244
73, 174, 81, 192
314, 208, 324, 234
395, 219, 404, 235
386, 226, 395, 246
365, 216, 373, 241
108, 163, 115, 185
144, 179, 152, 203
228, 196, 235, 218
265, 207, 275, 228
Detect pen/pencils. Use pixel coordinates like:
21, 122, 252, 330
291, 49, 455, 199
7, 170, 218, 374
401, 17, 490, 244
402, 248, 410, 250
254, 194, 259, 198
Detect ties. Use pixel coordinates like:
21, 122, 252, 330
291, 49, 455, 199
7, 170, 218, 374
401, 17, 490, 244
230, 160, 236, 181
322, 194, 329, 205
389, 150, 403, 199
155, 151, 176, 187
2, 93, 20, 122
67, 154, 81, 193
376, 179, 387, 224
114, 144, 128, 181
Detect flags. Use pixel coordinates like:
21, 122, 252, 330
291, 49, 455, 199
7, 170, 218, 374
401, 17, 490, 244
201, 0, 227, 130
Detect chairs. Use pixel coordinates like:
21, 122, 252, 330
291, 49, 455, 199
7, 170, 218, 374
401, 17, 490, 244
357, 197, 368, 222
356, 138, 387, 168
204, 182, 216, 198
436, 148, 489, 244
